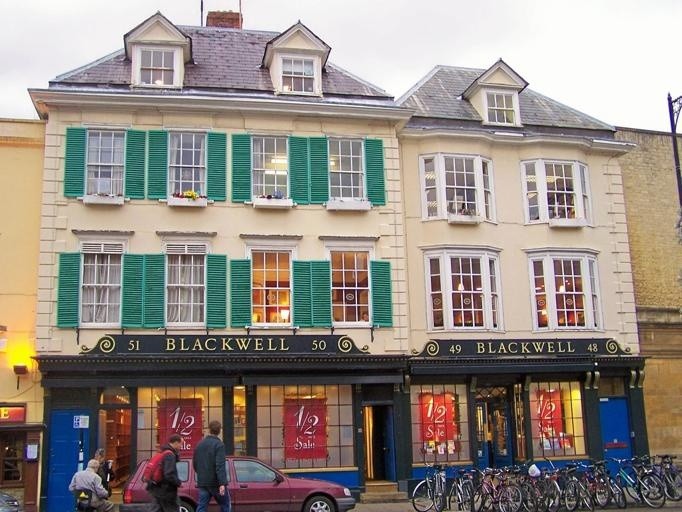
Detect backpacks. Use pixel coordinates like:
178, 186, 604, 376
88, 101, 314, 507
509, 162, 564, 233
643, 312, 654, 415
142, 450, 174, 487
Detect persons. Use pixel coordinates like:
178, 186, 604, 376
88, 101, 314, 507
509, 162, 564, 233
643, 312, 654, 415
151, 434, 184, 512
192, 420, 232, 512
68, 458, 115, 512
94, 448, 115, 497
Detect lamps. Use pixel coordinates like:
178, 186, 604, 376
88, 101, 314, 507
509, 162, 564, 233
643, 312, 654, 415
14, 365, 28, 375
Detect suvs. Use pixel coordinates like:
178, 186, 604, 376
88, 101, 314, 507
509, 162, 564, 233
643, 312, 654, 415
120, 456, 356, 512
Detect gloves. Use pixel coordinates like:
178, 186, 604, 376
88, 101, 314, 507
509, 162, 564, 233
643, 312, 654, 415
105, 467, 113, 474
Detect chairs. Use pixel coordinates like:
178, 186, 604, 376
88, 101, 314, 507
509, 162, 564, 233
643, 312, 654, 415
74, 489, 95, 512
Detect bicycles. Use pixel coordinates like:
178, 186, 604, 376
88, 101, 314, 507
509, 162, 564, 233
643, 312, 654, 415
412, 462, 447, 512
450, 455, 682, 512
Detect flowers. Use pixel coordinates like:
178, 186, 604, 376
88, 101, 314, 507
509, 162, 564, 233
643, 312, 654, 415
173, 190, 198, 200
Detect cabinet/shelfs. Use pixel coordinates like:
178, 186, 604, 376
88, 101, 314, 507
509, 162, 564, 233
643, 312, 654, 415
106, 398, 131, 488
